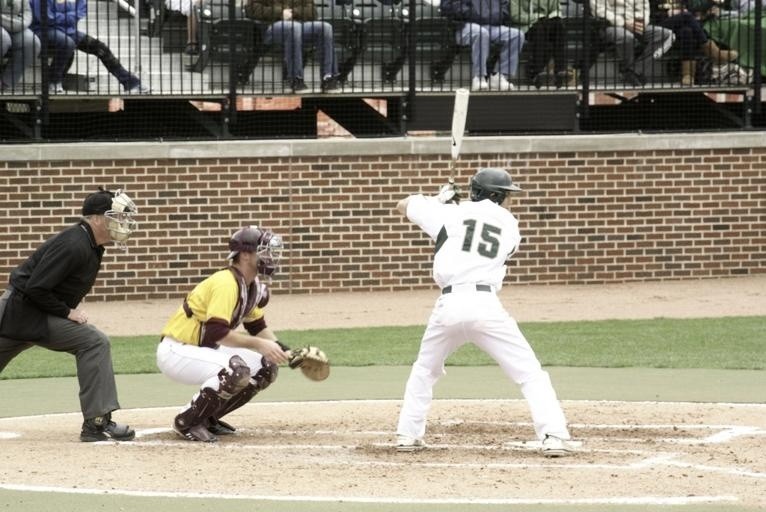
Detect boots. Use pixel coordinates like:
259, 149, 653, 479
681, 61, 695, 85
700, 40, 737, 68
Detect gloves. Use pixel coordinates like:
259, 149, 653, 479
437, 186, 461, 205
275, 341, 329, 380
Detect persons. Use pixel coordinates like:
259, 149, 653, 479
0, 0, 42, 90
510, 0, 575, 85
0, 186, 137, 442
29, 0, 154, 93
164, 0, 204, 55
244, 0, 341, 89
589, 0, 677, 86
649, 0, 739, 86
156, 225, 293, 443
395, 166, 575, 458
439, 0, 525, 92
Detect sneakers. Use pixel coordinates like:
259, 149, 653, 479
471, 76, 489, 91
171, 421, 219, 443
534, 72, 547, 89
291, 79, 309, 94
627, 69, 643, 86
210, 419, 235, 435
48, 82, 63, 93
487, 73, 514, 91
541, 434, 575, 457
79, 419, 134, 442
555, 70, 573, 88
185, 42, 200, 56
396, 435, 427, 453
321, 78, 340, 93
128, 83, 153, 95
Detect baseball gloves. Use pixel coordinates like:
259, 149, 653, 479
288, 343, 331, 381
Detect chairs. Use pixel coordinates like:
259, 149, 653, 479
399, 0, 445, 61
205, 0, 254, 64
316, 0, 352, 63
353, 1, 400, 63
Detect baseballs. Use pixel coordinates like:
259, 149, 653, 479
448, 87, 471, 191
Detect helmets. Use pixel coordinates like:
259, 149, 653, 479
469, 166, 522, 205
226, 226, 283, 285
83, 187, 137, 250
